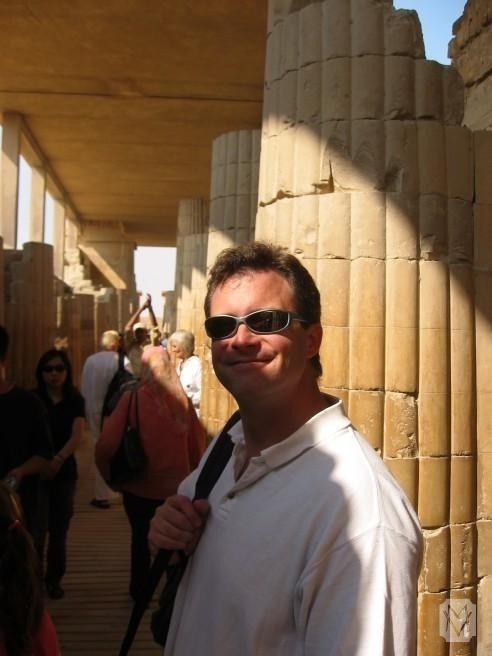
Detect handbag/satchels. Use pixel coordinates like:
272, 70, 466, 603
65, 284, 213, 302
107, 391, 148, 485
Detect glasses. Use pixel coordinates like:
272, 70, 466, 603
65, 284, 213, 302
43, 365, 64, 372
204, 309, 292, 340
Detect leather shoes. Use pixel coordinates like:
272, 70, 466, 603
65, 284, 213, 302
45, 575, 65, 600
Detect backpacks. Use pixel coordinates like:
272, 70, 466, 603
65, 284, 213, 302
149, 407, 242, 648
102, 352, 137, 417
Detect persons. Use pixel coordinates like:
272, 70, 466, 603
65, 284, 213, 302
146, 243, 424, 656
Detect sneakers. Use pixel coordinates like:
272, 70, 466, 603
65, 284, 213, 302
90, 498, 114, 509
